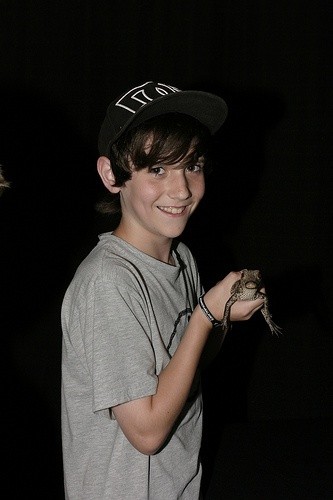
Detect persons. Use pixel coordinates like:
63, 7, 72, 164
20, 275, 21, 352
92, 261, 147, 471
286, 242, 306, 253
60, 81, 268, 500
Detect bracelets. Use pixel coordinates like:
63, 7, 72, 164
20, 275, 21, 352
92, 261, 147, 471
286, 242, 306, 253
199, 296, 221, 327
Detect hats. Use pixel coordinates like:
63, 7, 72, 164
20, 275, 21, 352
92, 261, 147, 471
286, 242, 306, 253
98, 80, 228, 158
